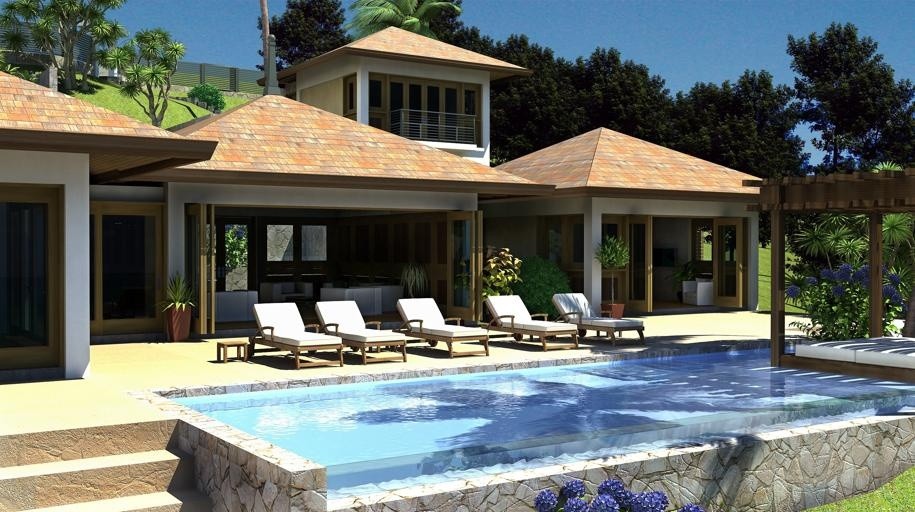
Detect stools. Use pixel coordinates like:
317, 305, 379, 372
217, 340, 249, 362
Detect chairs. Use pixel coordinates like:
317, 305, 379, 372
314, 300, 409, 365
551, 292, 645, 346
397, 296, 491, 359
487, 294, 580, 351
248, 302, 345, 368
681, 278, 713, 306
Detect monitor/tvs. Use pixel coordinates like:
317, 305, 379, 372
653, 247, 677, 267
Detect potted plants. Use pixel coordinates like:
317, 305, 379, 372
156, 272, 196, 342
591, 233, 632, 318
